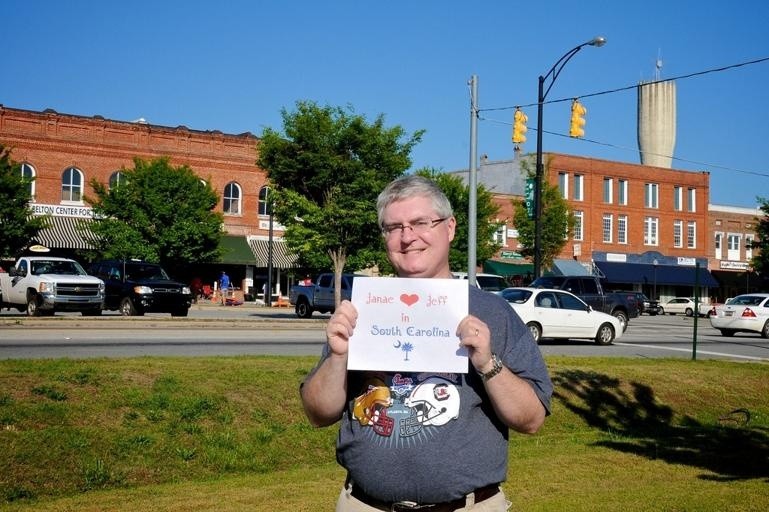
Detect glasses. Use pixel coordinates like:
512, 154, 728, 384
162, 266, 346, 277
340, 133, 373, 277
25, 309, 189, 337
382, 217, 446, 233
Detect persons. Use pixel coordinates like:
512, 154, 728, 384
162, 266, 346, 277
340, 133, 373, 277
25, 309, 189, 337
219, 271, 230, 306
298, 173, 554, 512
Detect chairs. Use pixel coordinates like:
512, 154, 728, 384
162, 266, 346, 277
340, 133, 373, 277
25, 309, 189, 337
540, 297, 552, 308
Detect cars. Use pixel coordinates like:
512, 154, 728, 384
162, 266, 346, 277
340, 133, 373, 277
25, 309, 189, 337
657, 293, 769, 337
451, 271, 624, 345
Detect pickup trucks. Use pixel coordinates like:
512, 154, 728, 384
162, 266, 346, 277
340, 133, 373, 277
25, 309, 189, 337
0, 256, 106, 317
289, 273, 372, 318
529, 274, 636, 333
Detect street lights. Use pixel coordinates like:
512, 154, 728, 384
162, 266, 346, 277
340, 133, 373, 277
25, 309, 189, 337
534, 38, 607, 280
267, 197, 274, 306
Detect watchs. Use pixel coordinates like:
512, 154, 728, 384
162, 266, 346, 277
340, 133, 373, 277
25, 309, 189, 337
477, 354, 503, 380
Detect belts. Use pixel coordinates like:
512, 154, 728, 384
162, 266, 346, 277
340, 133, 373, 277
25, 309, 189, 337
351, 484, 500, 512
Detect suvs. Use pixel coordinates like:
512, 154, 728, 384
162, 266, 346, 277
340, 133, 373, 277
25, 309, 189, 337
85, 261, 195, 316
613, 289, 659, 316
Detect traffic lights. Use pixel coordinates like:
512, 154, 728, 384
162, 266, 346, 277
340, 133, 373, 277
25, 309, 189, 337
514, 110, 529, 145
568, 102, 587, 138
